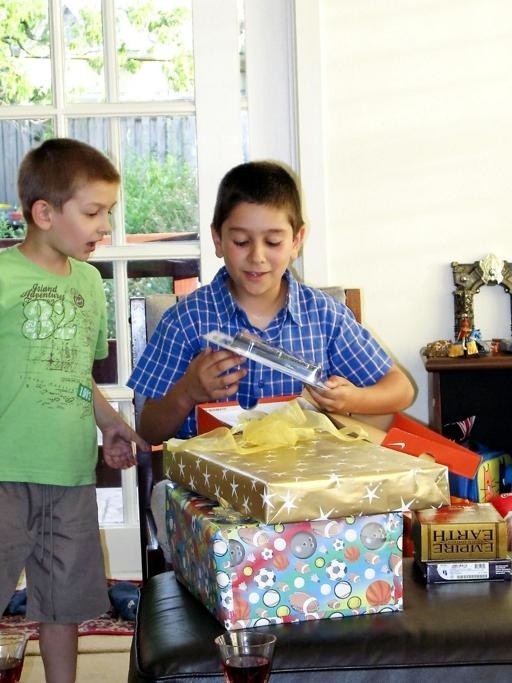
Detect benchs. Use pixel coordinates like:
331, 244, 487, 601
130, 555, 511, 682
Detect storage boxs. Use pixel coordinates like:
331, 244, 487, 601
163, 484, 409, 630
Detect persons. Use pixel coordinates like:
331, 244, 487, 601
120, 157, 418, 445
0, 136, 151, 683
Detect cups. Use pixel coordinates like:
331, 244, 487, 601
215, 632, 277, 683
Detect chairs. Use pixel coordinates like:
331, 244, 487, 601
136, 284, 363, 558
